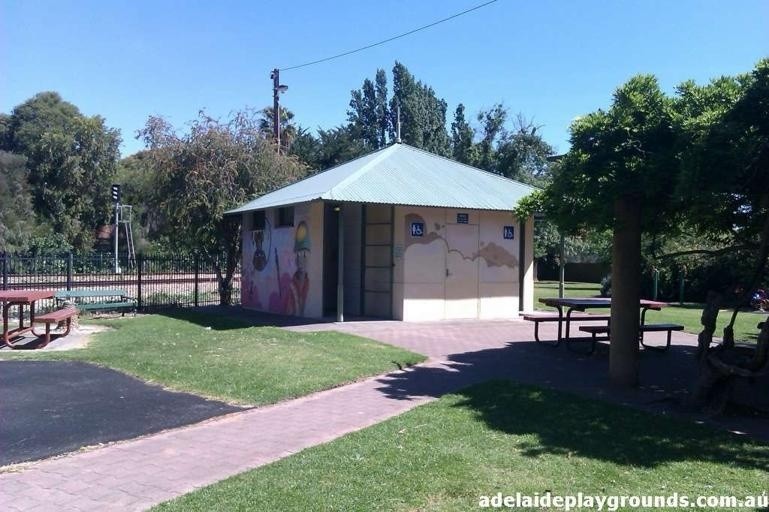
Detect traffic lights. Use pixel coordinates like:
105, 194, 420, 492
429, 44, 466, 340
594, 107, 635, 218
110, 184, 121, 203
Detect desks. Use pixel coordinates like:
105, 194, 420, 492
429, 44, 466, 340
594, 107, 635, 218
1, 290, 53, 348
538, 297, 668, 353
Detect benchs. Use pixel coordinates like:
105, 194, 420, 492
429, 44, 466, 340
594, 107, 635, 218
35, 308, 80, 350
56, 288, 136, 320
524, 314, 684, 356
